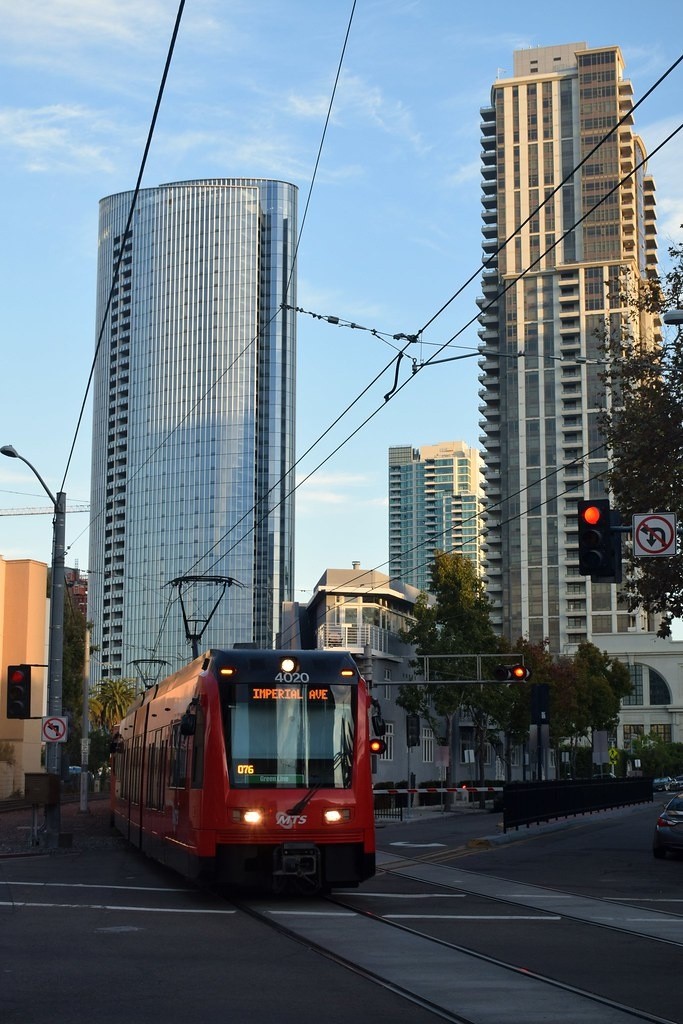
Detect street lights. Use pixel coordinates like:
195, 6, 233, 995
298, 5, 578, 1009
0, 445, 64, 853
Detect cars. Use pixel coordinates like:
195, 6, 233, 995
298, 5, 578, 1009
592, 773, 616, 779
495, 793, 505, 810
653, 776, 671, 791
652, 790, 683, 860
665, 776, 682, 789
70, 767, 94, 782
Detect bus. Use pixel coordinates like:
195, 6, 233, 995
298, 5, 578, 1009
110, 649, 386, 902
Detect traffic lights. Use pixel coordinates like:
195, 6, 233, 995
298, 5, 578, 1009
577, 499, 612, 577
7, 665, 33, 720
494, 666, 529, 681
369, 741, 388, 757
